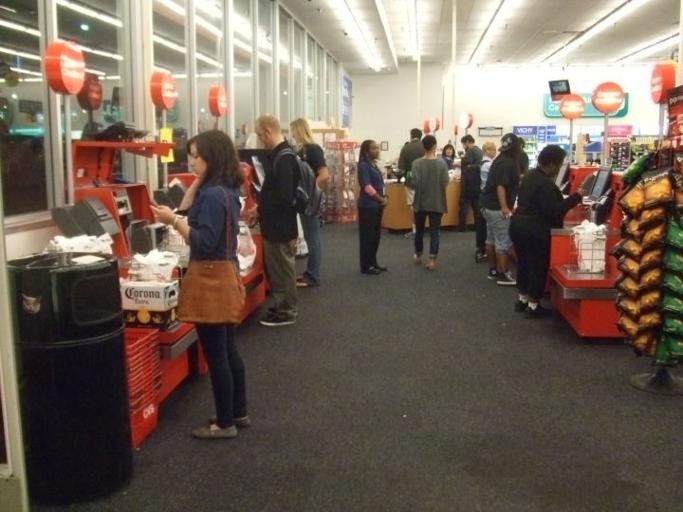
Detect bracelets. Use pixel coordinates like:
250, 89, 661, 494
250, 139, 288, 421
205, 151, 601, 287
172, 216, 179, 230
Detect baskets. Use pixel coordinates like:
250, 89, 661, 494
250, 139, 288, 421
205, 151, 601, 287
123, 327, 162, 448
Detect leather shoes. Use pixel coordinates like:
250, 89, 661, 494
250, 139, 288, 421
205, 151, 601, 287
208, 414, 249, 428
376, 264, 387, 272
425, 263, 435, 270
514, 300, 529, 312
362, 266, 382, 275
525, 304, 551, 318
192, 424, 237, 439
413, 254, 420, 264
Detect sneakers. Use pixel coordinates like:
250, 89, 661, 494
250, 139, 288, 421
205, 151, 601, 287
487, 268, 511, 280
259, 316, 295, 327
296, 278, 310, 286
496, 272, 516, 285
267, 307, 296, 317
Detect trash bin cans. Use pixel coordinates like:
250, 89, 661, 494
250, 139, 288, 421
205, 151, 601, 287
5, 252, 133, 506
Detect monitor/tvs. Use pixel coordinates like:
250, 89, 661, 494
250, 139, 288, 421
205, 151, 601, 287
548, 79, 571, 95
589, 167, 612, 200
555, 162, 570, 187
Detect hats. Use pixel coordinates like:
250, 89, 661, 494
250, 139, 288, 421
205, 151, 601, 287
498, 134, 519, 153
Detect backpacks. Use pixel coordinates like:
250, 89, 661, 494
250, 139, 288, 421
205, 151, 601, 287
272, 148, 324, 220
462, 160, 488, 200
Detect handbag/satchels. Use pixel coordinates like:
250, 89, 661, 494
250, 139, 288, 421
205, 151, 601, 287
176, 260, 246, 325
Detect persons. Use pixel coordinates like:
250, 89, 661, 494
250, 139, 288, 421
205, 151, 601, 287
474, 141, 496, 263
289, 117, 329, 287
356, 139, 388, 276
516, 137, 529, 174
254, 114, 315, 326
397, 127, 456, 271
508, 145, 587, 319
452, 134, 483, 232
481, 133, 520, 285
148, 129, 250, 439
